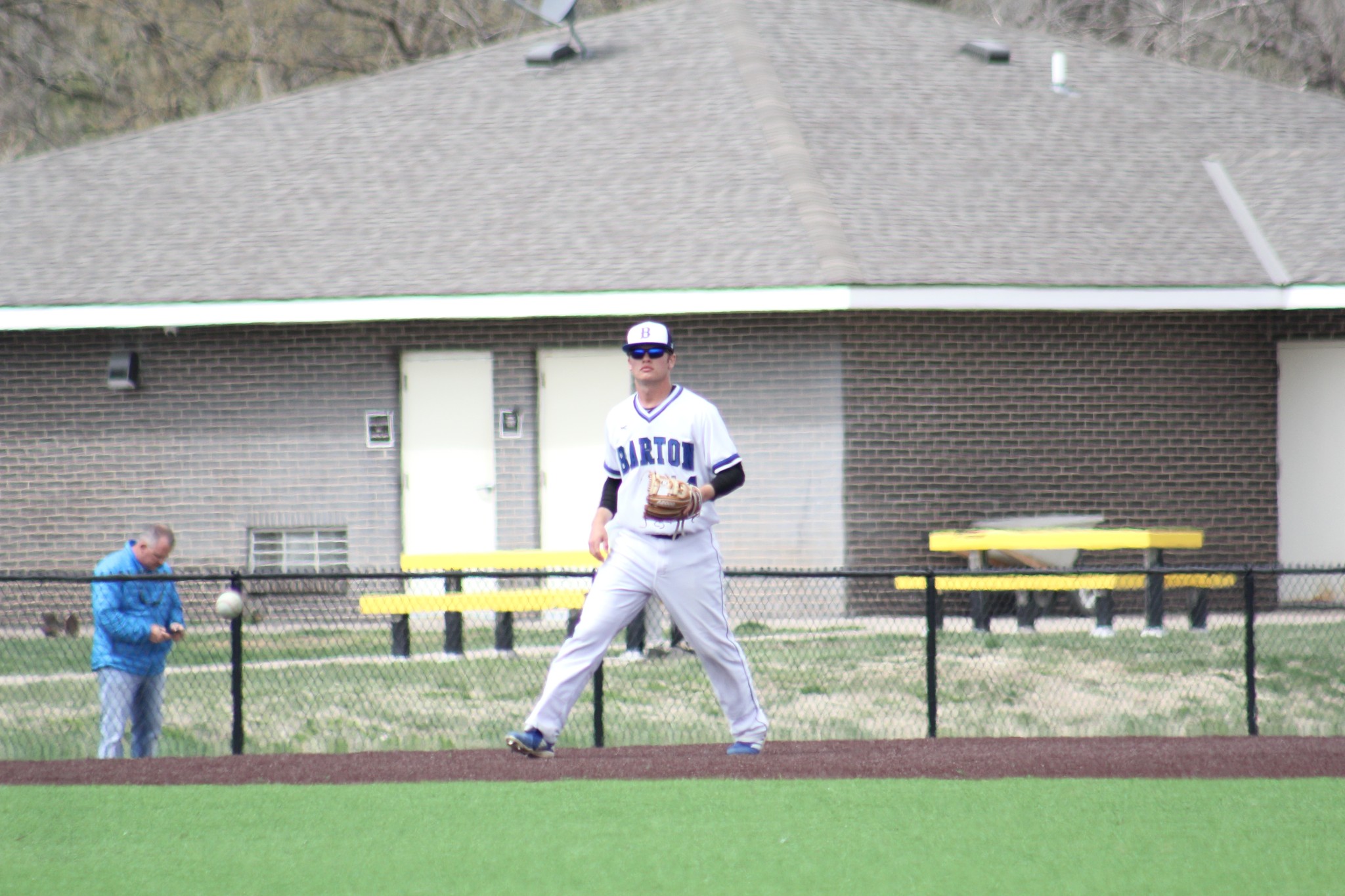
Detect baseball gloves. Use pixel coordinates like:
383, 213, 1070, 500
639, 467, 703, 541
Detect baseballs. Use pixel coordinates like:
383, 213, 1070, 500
215, 591, 242, 619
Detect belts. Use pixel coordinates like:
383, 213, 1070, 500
647, 534, 683, 540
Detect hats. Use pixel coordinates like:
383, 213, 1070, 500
622, 320, 674, 353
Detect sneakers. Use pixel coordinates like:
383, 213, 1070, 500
506, 728, 555, 758
726, 734, 766, 755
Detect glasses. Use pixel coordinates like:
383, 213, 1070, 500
628, 348, 671, 360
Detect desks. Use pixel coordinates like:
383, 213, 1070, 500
927, 526, 1205, 629
401, 546, 607, 654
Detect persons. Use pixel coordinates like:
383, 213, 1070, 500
506, 323, 770, 756
90, 523, 186, 760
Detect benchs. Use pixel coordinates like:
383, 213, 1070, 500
359, 589, 586, 656
892, 574, 1237, 628
